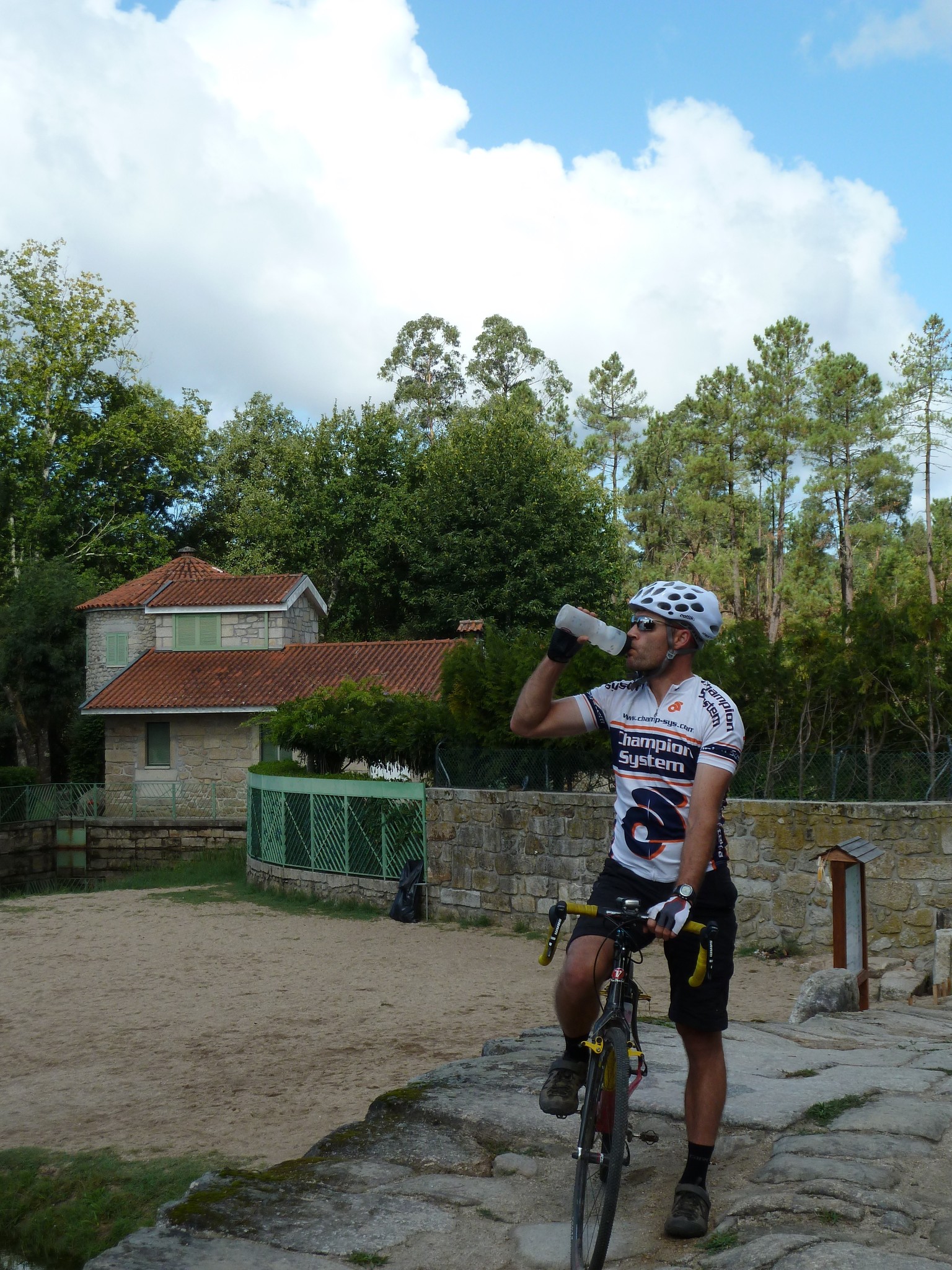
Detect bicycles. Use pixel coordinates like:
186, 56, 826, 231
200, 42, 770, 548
538, 897, 717, 1270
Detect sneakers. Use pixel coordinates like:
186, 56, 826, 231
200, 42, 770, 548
538, 1056, 590, 1116
664, 1183, 711, 1239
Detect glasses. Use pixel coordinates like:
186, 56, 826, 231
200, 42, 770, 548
630, 614, 685, 631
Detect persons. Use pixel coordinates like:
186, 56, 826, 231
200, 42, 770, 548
510, 581, 745, 1241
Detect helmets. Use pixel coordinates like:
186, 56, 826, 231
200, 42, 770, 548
628, 580, 722, 651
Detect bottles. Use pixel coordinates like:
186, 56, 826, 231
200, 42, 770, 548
555, 604, 632, 657
624, 995, 632, 1024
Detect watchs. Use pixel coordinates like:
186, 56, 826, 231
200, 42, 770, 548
671, 884, 696, 906
